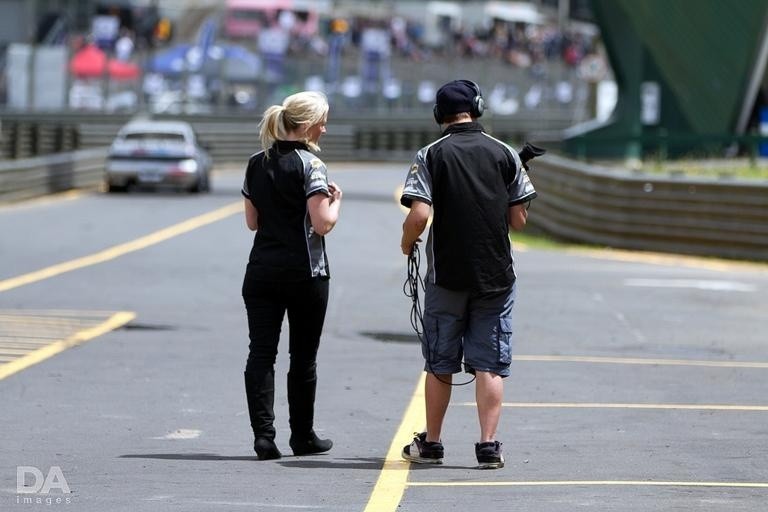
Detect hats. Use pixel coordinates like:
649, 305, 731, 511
435, 80, 488, 115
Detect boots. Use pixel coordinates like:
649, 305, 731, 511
244, 369, 282, 459
288, 368, 333, 457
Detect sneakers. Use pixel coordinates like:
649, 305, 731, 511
474, 440, 503, 469
402, 431, 446, 465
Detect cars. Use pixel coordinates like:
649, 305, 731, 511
104, 120, 214, 193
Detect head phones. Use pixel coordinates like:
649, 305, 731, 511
433, 79, 485, 125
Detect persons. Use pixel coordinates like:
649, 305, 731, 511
238, 90, 342, 459
399, 79, 538, 468
